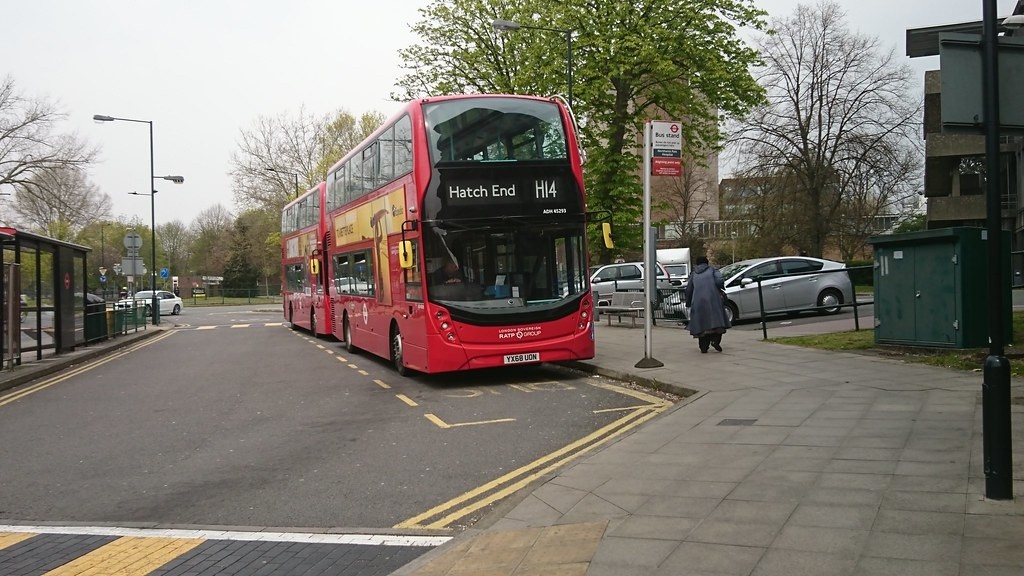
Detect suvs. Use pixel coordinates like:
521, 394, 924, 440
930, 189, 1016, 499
565, 260, 673, 314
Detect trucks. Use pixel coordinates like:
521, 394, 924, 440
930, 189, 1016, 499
657, 248, 693, 292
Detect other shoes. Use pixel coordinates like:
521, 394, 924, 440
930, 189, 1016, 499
711, 341, 722, 351
702, 349, 707, 353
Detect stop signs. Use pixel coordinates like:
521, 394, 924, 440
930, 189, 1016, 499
64, 272, 70, 289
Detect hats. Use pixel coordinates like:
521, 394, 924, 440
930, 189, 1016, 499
696, 256, 708, 265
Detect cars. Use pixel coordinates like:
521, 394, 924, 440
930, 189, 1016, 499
662, 256, 855, 327
115, 290, 184, 318
0, 290, 30, 323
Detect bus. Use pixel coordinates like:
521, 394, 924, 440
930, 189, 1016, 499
277, 93, 620, 376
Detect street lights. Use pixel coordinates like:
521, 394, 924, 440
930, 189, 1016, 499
266, 167, 298, 198
100, 223, 112, 282
491, 20, 572, 111
92, 115, 184, 323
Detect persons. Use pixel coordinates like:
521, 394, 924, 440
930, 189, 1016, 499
432, 254, 466, 284
685, 257, 732, 353
